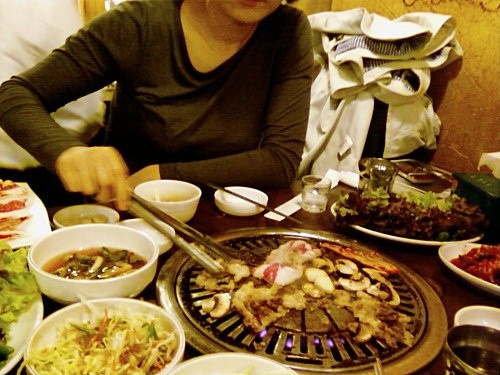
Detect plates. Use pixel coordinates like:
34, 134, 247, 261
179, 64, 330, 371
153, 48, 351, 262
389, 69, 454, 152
167, 352, 303, 375
0, 250, 45, 375
211, 186, 269, 217
439, 245, 500, 299
330, 188, 486, 245
118, 216, 176, 257
52, 204, 121, 229
454, 305, 500, 339
0, 178, 52, 252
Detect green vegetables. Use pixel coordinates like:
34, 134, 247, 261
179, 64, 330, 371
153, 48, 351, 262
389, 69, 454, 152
334, 187, 486, 240
0, 241, 40, 371
52, 247, 142, 279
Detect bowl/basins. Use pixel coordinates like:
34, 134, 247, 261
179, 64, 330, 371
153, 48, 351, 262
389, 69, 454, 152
28, 223, 158, 306
132, 180, 202, 222
442, 324, 500, 375
20, 294, 185, 375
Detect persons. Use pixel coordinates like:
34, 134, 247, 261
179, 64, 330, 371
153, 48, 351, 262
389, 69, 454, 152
0, 0, 314, 212
0, 0, 106, 209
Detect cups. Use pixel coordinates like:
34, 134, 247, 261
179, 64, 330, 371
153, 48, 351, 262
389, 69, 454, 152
357, 158, 399, 192
301, 174, 332, 213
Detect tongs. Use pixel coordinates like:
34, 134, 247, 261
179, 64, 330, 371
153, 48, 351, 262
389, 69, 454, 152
109, 185, 268, 279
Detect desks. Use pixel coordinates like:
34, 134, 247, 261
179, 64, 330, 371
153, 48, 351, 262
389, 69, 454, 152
0, 180, 500, 375
